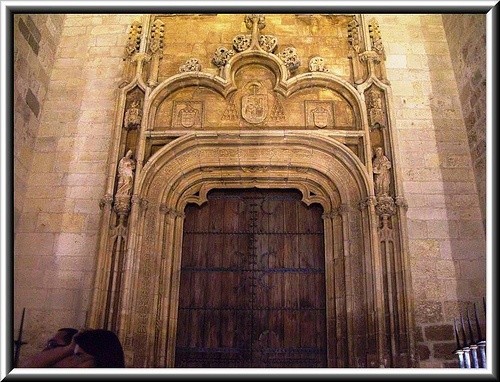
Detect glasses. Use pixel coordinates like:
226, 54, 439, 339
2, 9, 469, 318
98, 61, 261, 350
45, 342, 64, 349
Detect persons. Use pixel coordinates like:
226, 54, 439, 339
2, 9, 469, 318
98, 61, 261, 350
41, 328, 78, 368
115, 150, 135, 196
371, 146, 391, 196
20, 329, 125, 368
